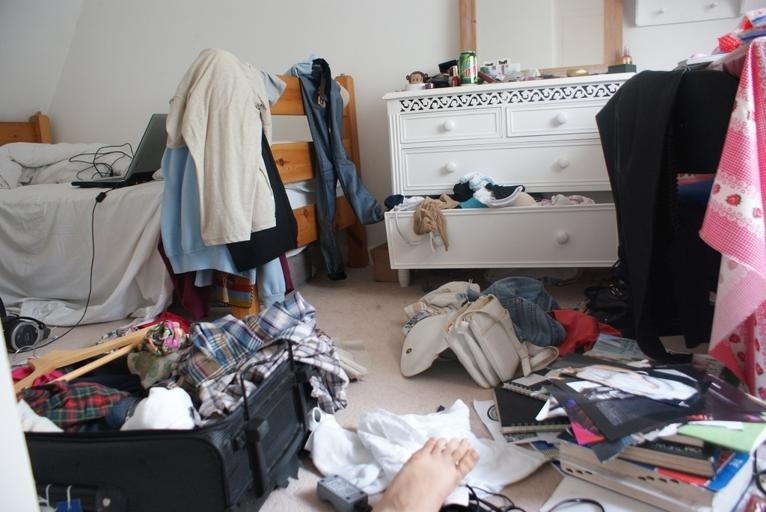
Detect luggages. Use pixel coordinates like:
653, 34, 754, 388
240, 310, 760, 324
23, 337, 317, 507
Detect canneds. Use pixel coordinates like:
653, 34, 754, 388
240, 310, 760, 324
459, 51, 478, 85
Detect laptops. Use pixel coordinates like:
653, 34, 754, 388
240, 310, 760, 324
71, 113, 168, 189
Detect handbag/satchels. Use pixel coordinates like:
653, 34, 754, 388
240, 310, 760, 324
400, 292, 560, 387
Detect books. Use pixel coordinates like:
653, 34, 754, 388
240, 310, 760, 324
493, 376, 766, 512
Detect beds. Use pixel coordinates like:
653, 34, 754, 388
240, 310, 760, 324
0, 75, 368, 321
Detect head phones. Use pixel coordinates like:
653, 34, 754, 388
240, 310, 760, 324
4, 316, 51, 351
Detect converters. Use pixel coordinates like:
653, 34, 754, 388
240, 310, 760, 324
316, 475, 373, 512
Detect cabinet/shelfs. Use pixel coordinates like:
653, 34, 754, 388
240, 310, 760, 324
384, 69, 637, 289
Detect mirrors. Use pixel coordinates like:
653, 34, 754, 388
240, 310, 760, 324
457, 1, 623, 79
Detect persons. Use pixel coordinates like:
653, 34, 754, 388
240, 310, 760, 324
370, 436, 480, 512
